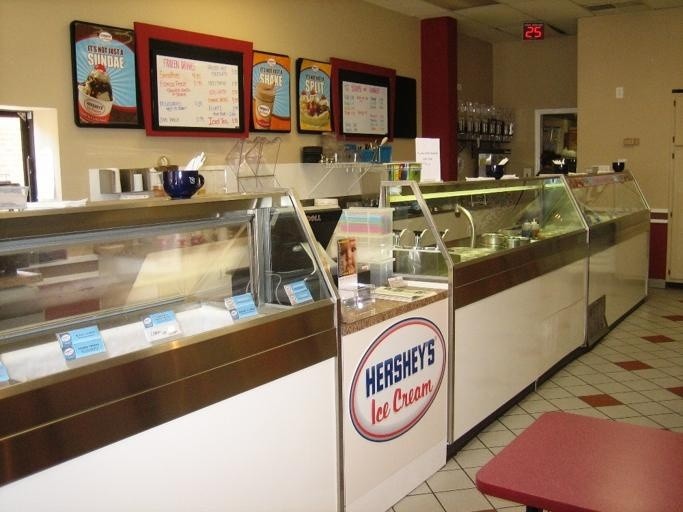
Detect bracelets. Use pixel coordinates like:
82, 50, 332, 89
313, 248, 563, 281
478, 232, 530, 251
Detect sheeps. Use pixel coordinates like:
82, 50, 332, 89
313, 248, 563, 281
455, 204, 475, 250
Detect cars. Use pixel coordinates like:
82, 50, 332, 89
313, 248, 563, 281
252, 93, 275, 129
553, 164, 567, 174
161, 169, 204, 202
611, 162, 625, 172
484, 163, 505, 179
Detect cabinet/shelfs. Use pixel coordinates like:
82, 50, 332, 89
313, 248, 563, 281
520, 216, 539, 238
457, 101, 515, 136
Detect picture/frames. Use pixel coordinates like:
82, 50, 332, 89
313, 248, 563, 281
475, 410, 683, 512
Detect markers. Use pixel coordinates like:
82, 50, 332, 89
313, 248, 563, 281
307, 87, 319, 117
255, 81, 276, 103
77, 64, 114, 102
300, 90, 309, 115
319, 94, 329, 117
